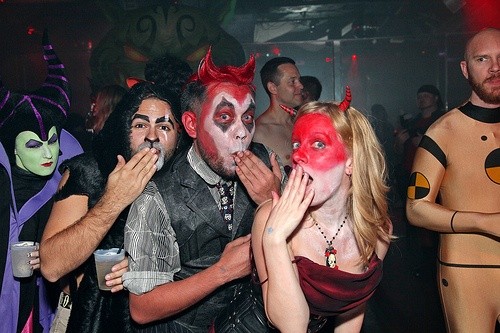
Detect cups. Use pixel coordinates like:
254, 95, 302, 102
11, 241, 35, 277
93, 248, 125, 291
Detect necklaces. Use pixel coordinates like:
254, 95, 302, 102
306, 208, 350, 268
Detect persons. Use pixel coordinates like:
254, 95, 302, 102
0, 24, 449, 333
406, 31, 500, 333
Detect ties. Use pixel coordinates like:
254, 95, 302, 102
216, 180, 234, 234
